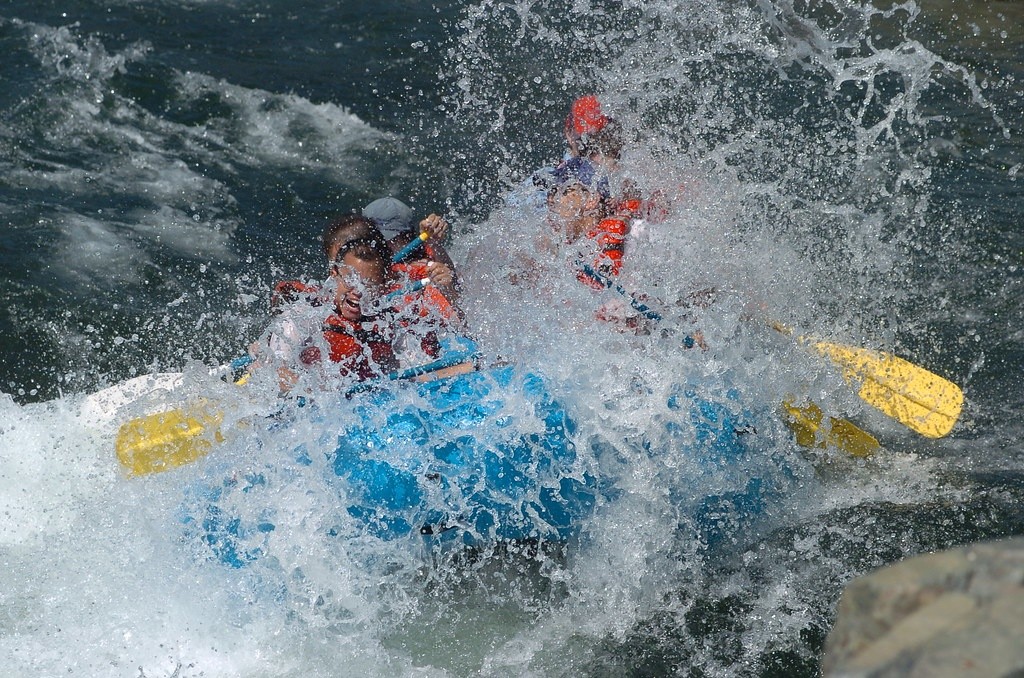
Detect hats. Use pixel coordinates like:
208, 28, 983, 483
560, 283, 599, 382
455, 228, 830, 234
568, 95, 610, 136
532, 157, 610, 198
362, 196, 413, 242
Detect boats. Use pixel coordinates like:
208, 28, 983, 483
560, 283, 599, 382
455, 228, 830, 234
181, 350, 769, 594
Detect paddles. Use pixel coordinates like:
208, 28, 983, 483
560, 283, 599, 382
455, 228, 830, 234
116, 352, 470, 479
573, 258, 880, 455
195, 233, 430, 407
740, 312, 964, 437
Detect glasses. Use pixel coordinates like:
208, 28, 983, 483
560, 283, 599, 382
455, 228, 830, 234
336, 271, 378, 290
547, 187, 588, 196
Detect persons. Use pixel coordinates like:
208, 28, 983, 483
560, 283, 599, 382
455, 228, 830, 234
505, 95, 768, 352
247, 196, 474, 395
